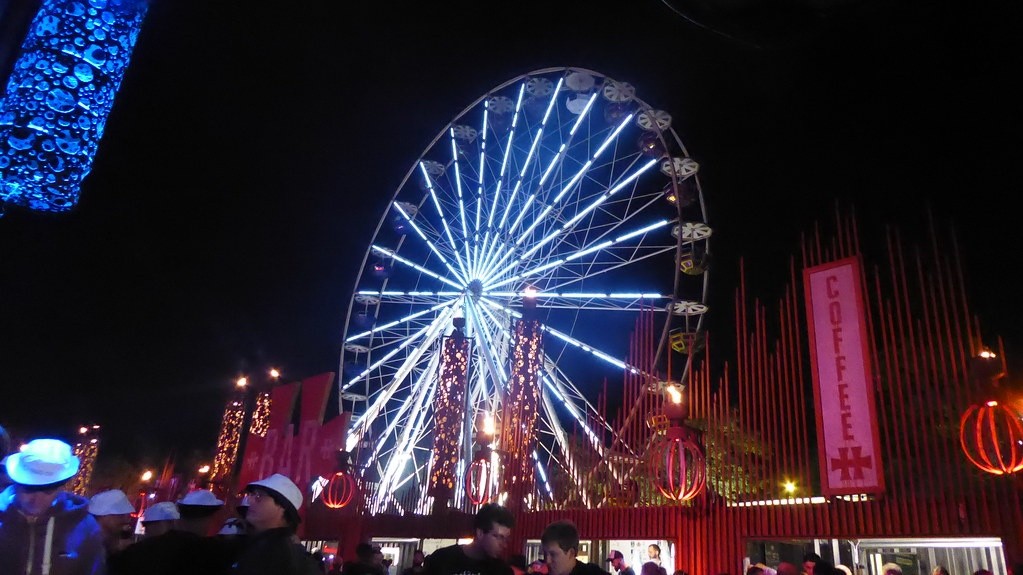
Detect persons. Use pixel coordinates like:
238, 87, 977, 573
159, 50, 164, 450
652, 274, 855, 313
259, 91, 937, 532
208, 473, 326, 575
606, 550, 635, 575
313, 542, 424, 575
108, 490, 227, 575
746, 553, 993, 575
421, 503, 515, 575
0, 439, 106, 575
541, 520, 612, 575
142, 502, 181, 541
643, 545, 662, 566
87, 489, 135, 561
640, 562, 689, 575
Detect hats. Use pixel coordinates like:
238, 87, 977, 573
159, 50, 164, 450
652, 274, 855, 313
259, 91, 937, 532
178, 490, 223, 506
606, 551, 623, 561
249, 473, 304, 523
236, 495, 249, 514
141, 503, 180, 521
87, 489, 137, 516
6, 438, 80, 486
